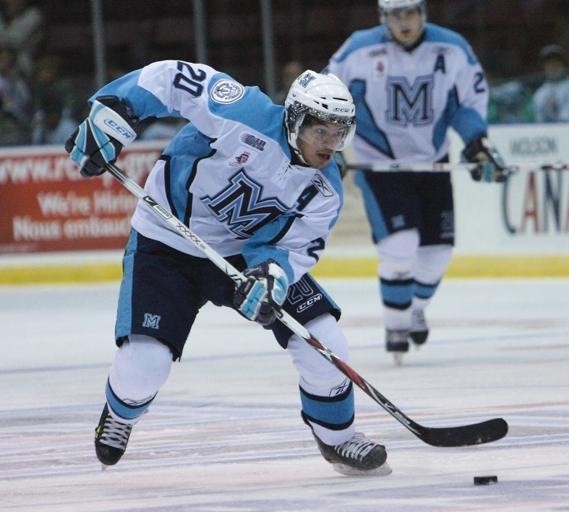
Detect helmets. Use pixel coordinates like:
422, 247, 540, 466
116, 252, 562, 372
284, 69, 357, 148
537, 44, 568, 66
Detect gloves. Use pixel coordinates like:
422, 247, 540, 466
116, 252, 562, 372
231, 260, 288, 326
459, 134, 510, 183
64, 98, 139, 178
335, 151, 347, 178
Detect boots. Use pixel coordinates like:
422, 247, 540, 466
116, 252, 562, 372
310, 429, 387, 470
383, 306, 429, 354
94, 405, 134, 465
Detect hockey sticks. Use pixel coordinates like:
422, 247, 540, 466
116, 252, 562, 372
104, 162, 508, 448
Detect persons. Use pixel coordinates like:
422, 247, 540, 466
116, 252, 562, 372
0, 0, 194, 146
320, 0, 509, 353
61, 58, 387, 473
279, 43, 309, 105
481, 40, 569, 125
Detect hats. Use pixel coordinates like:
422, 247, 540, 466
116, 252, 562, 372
378, 0, 427, 21
